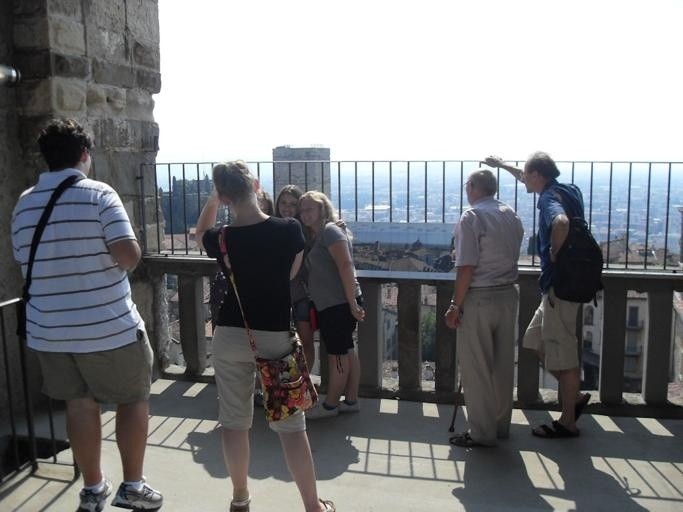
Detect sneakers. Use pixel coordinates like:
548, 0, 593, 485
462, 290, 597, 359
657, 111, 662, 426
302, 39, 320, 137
450, 432, 481, 446
306, 401, 360, 419
80, 481, 111, 512
319, 499, 335, 512
112, 483, 162, 509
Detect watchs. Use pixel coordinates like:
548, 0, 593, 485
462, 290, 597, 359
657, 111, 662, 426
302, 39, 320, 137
448, 304, 460, 311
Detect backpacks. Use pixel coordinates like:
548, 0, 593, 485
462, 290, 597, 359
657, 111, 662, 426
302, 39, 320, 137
547, 185, 603, 308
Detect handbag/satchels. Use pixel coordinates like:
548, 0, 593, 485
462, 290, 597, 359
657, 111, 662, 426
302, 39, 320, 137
256, 340, 319, 420
16, 301, 26, 339
310, 305, 318, 331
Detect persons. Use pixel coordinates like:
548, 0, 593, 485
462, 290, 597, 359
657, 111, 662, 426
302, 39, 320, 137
193, 158, 340, 512
481, 150, 593, 440
9, 116, 165, 512
256, 188, 275, 411
275, 183, 347, 378
298, 189, 368, 423
443, 167, 525, 450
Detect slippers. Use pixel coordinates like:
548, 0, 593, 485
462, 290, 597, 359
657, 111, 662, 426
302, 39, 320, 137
533, 392, 590, 437
